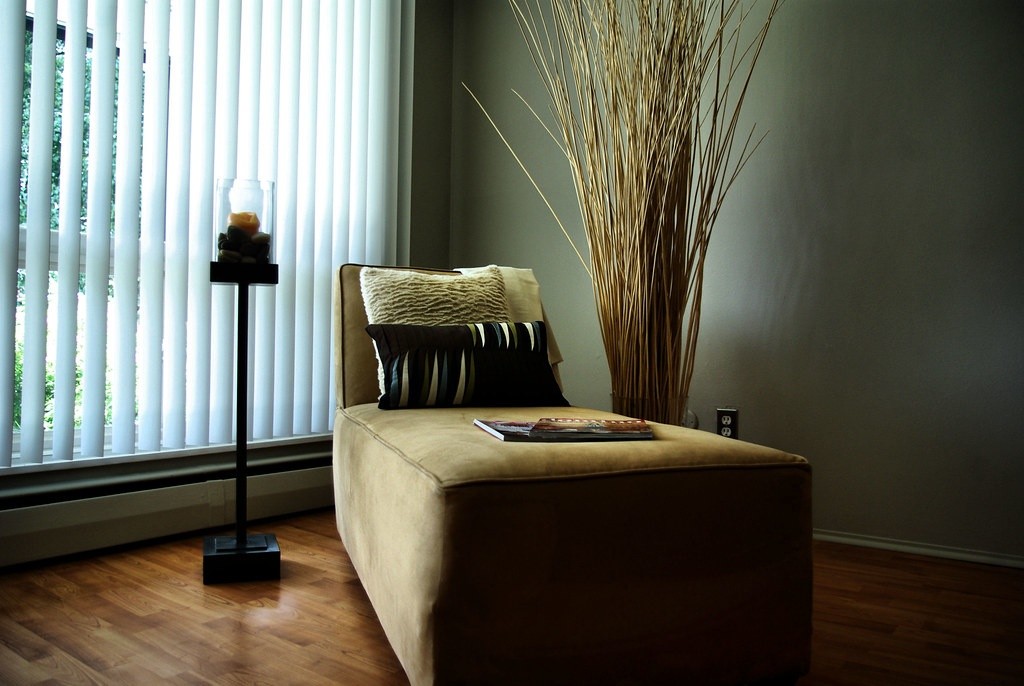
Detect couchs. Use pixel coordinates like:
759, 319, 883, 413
332, 262, 816, 686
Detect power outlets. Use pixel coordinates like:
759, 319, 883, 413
714, 409, 737, 438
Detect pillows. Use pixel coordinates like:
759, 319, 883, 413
360, 267, 570, 408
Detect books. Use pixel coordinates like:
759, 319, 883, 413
473, 417, 653, 441
